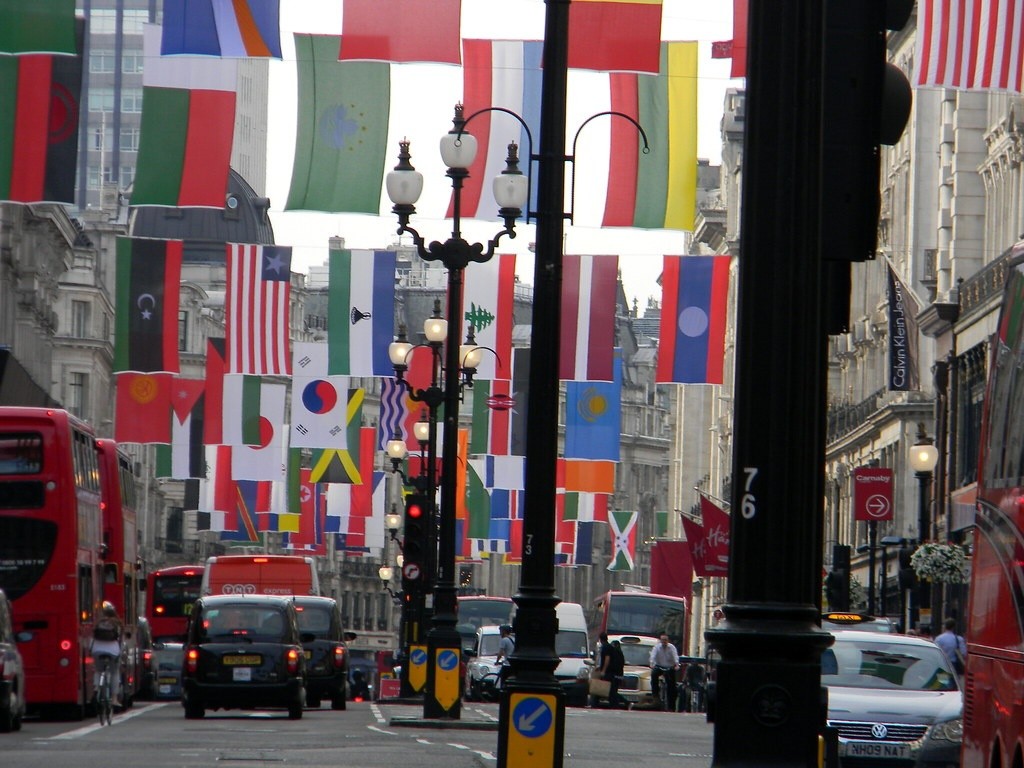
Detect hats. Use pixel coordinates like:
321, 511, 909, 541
499, 624, 511, 632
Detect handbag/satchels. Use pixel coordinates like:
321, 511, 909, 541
955, 648, 965, 675
590, 679, 611, 697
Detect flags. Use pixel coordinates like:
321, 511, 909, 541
600, 40, 696, 233
160, 0, 283, 59
284, 33, 391, 216
729, 0, 748, 78
540, 0, 663, 75
912, 0, 1024, 92
651, 495, 729, 611
1, 19, 86, 205
337, 0, 462, 67
113, 236, 638, 572
887, 266, 920, 391
444, 39, 544, 224
0, 0, 76, 56
655, 254, 732, 384
128, 24, 238, 209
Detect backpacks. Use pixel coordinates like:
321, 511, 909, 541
93, 617, 121, 640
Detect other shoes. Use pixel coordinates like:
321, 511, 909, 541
110, 700, 121, 706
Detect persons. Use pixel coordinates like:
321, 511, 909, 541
495, 625, 514, 687
585, 632, 634, 710
649, 634, 707, 712
908, 624, 934, 642
936, 620, 966, 685
90, 606, 124, 707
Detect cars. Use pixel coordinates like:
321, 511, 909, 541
0, 588, 35, 730
583, 634, 661, 709
141, 642, 185, 701
181, 591, 381, 720
819, 609, 964, 768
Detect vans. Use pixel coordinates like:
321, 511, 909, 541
464, 625, 512, 701
198, 553, 322, 629
554, 602, 596, 708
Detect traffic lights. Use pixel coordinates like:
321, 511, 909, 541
405, 492, 429, 561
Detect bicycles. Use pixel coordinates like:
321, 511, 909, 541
97, 634, 130, 727
479, 662, 505, 702
651, 666, 680, 712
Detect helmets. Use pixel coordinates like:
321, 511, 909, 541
102, 600, 115, 610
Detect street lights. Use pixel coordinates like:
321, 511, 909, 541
388, 296, 482, 703
383, 408, 441, 667
386, 101, 528, 720
909, 422, 939, 604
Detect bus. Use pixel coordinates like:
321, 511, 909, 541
1, 406, 119, 719
456, 594, 513, 696
587, 590, 691, 682
96, 437, 148, 708
143, 563, 205, 643
962, 240, 1024, 768
